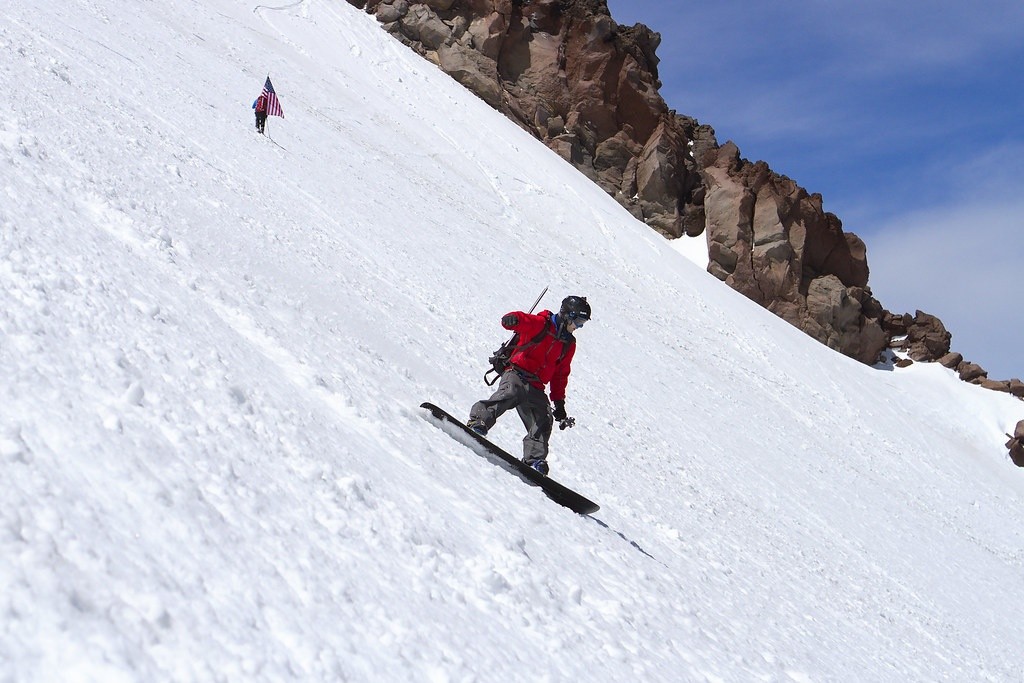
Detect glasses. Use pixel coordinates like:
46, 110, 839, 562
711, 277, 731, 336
569, 316, 585, 327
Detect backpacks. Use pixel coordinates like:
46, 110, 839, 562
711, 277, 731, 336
256, 96, 267, 113
484, 319, 570, 386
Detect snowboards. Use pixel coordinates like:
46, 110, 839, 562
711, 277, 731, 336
420, 401, 601, 515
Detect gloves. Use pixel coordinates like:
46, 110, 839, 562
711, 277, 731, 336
552, 399, 566, 421
502, 315, 518, 326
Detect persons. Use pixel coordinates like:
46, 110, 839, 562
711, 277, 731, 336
252, 96, 266, 132
466, 295, 592, 476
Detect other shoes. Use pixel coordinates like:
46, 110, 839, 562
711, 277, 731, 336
257, 126, 264, 132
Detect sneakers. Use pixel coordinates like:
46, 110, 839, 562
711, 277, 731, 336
468, 424, 486, 436
531, 458, 549, 474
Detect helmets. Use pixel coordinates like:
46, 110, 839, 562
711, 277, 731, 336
562, 296, 591, 321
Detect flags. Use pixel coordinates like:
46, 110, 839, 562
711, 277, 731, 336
261, 77, 285, 119
